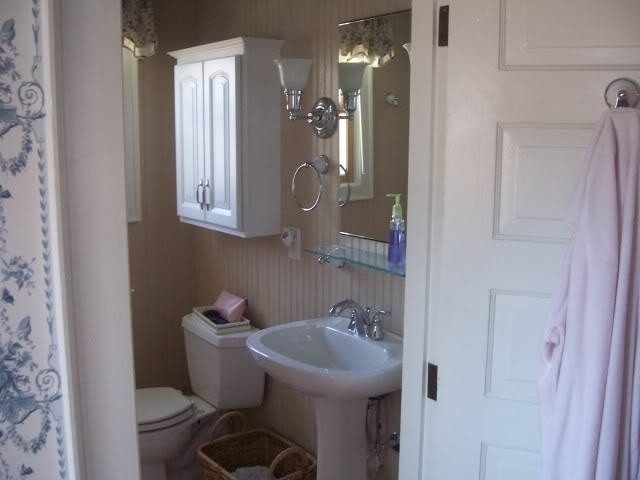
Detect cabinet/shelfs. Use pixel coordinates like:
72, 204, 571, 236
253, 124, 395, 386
160, 33, 289, 241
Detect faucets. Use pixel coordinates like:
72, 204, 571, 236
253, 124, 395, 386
328, 299, 372, 338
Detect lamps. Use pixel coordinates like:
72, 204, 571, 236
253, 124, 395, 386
330, 62, 372, 121
269, 56, 340, 143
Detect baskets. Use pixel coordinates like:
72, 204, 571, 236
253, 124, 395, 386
194, 409, 318, 480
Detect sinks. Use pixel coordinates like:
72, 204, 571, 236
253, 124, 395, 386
246, 315, 403, 399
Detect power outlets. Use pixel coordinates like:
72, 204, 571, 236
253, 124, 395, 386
286, 224, 303, 263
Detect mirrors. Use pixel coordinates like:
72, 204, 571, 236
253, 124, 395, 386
325, 5, 418, 250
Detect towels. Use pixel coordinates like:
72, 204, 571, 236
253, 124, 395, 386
539, 102, 639, 480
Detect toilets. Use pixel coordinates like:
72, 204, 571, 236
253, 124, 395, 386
135, 312, 266, 480
